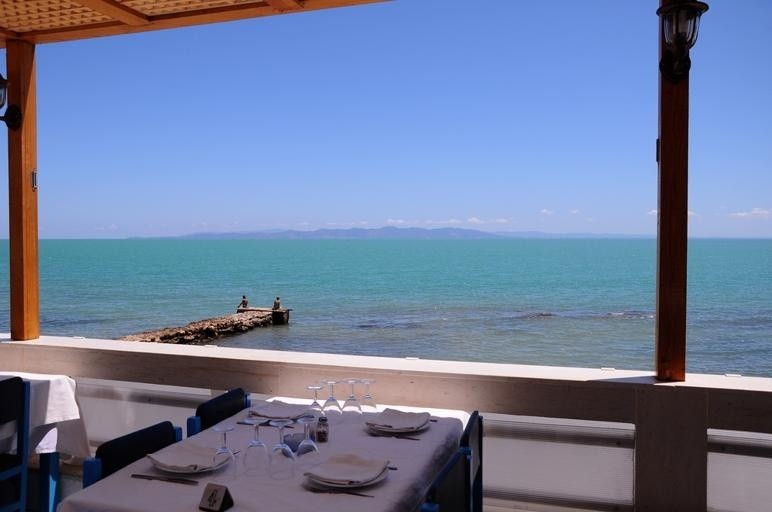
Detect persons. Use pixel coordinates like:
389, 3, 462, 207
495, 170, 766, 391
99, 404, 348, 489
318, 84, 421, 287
272, 296, 282, 310
237, 295, 249, 308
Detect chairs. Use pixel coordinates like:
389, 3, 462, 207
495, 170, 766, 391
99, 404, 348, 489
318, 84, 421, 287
420, 410, 484, 512
82, 388, 252, 488
0, 377, 30, 509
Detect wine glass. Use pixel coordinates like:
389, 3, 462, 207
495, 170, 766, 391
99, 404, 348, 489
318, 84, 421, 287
211, 418, 319, 479
304, 378, 378, 424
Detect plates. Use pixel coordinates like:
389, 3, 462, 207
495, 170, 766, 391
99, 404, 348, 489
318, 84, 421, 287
249, 410, 304, 421
310, 472, 392, 488
153, 458, 230, 474
368, 423, 427, 433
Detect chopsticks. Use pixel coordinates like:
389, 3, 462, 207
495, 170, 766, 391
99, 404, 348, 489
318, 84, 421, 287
131, 473, 199, 486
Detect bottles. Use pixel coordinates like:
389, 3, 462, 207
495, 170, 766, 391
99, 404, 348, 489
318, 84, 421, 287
316, 417, 329, 444
